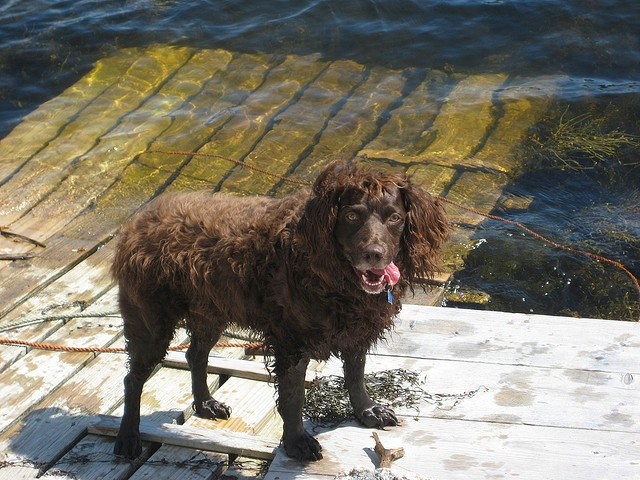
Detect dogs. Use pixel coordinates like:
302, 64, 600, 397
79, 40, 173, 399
106, 158, 451, 463
